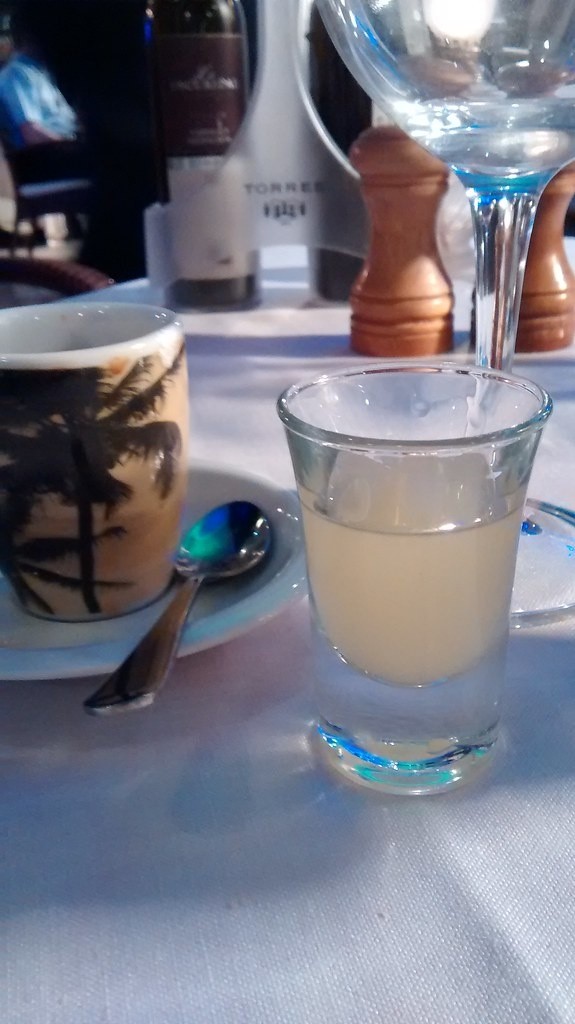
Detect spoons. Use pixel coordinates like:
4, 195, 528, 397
82, 499, 274, 715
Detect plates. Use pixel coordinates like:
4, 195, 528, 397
0, 458, 310, 682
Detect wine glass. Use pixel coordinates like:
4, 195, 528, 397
314, 0, 575, 629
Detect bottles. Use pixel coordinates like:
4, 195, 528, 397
138, 0, 262, 316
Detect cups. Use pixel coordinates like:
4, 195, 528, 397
1, 301, 189, 623
276, 361, 575, 797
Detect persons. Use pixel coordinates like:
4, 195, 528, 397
2, 0, 157, 283
